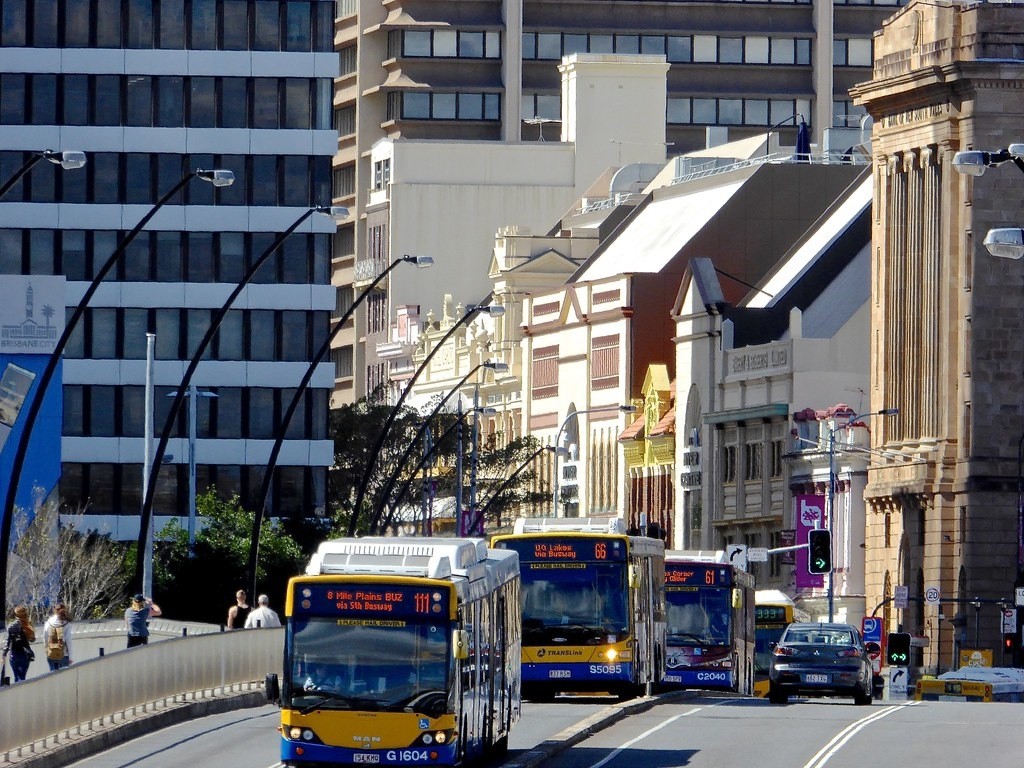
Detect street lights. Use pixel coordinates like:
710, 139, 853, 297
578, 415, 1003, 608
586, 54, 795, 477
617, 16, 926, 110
552, 405, 636, 519
243, 252, 437, 629
0, 168, 237, 633
365, 358, 509, 536
132, 206, 352, 643
344, 302, 508, 538
828, 408, 900, 622
464, 443, 568, 537
378, 405, 496, 535
995, 597, 1012, 668
970, 597, 984, 649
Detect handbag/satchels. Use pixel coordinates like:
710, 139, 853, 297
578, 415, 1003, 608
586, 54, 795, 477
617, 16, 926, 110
23, 647, 35, 662
1, 664, 10, 687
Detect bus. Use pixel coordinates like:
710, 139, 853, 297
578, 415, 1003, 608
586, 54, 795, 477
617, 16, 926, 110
753, 589, 811, 702
489, 515, 670, 703
658, 548, 758, 698
262, 534, 524, 768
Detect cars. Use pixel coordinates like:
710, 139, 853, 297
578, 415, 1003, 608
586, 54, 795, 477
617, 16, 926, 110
767, 621, 873, 706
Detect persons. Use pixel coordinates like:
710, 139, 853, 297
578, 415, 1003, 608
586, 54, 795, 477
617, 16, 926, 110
303, 664, 343, 693
43, 604, 74, 671
0, 605, 36, 687
243, 595, 281, 627
125, 595, 162, 648
228, 590, 256, 630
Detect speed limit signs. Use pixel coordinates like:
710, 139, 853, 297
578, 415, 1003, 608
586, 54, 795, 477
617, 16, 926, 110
925, 586, 941, 605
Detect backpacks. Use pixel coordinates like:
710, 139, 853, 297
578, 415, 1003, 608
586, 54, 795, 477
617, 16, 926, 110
47, 622, 68, 661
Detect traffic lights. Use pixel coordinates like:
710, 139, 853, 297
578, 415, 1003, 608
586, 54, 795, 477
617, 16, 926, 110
1004, 634, 1012, 654
887, 632, 911, 667
807, 529, 833, 575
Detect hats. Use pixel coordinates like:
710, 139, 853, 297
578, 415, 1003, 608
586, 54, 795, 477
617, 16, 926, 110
134, 594, 145, 603
